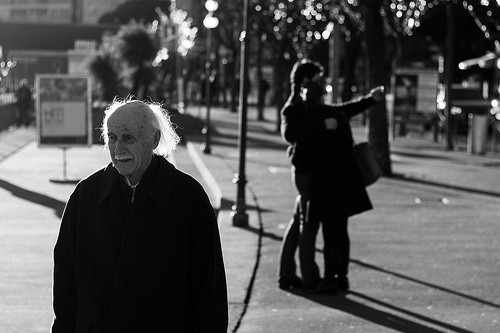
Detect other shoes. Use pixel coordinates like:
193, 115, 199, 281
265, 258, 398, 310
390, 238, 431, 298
278, 274, 349, 293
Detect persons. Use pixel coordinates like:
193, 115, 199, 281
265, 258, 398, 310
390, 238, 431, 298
277, 60, 385, 295
15, 79, 32, 128
52, 98, 229, 333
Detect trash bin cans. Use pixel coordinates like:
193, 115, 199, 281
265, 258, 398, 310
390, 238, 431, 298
466, 113, 490, 157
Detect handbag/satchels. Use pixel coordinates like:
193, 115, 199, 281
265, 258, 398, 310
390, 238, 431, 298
353, 141, 388, 187
